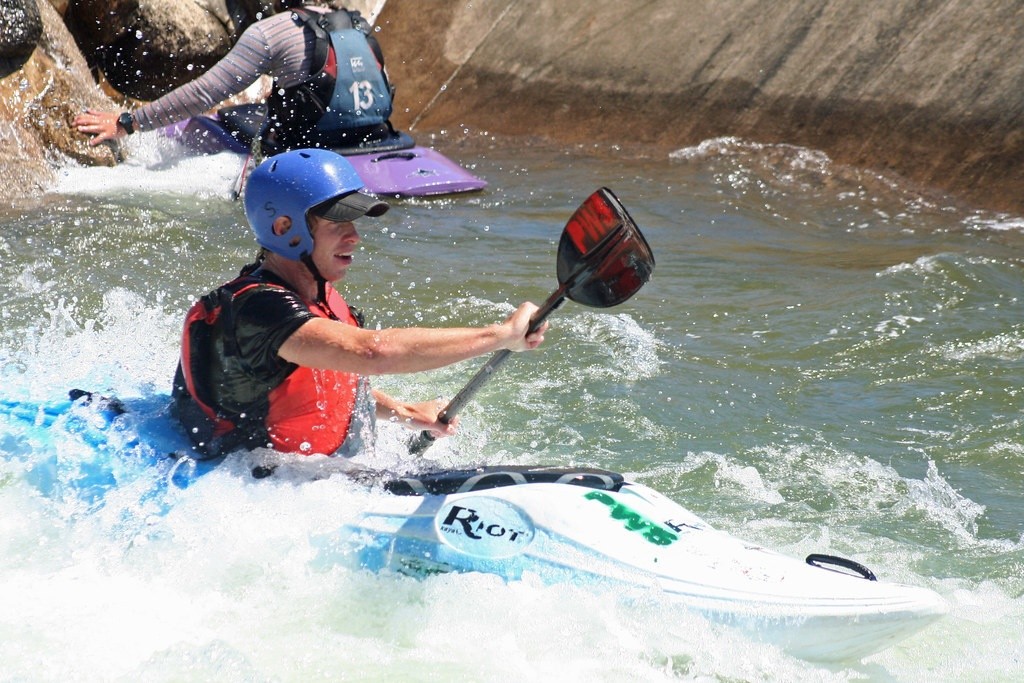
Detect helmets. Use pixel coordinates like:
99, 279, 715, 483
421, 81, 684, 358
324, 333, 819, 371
244, 148, 363, 261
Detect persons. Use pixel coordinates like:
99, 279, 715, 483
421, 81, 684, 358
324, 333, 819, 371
172, 148, 549, 457
72, 0, 396, 147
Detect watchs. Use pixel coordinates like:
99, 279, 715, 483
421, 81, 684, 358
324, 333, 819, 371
118, 112, 134, 134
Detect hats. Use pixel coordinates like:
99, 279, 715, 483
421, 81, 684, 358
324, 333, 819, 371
306, 191, 391, 223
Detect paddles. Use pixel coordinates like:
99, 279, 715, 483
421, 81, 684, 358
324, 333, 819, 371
409, 185, 655, 455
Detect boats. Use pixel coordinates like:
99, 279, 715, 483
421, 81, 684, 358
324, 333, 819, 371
0, 386, 953, 668
47, 60, 490, 201
